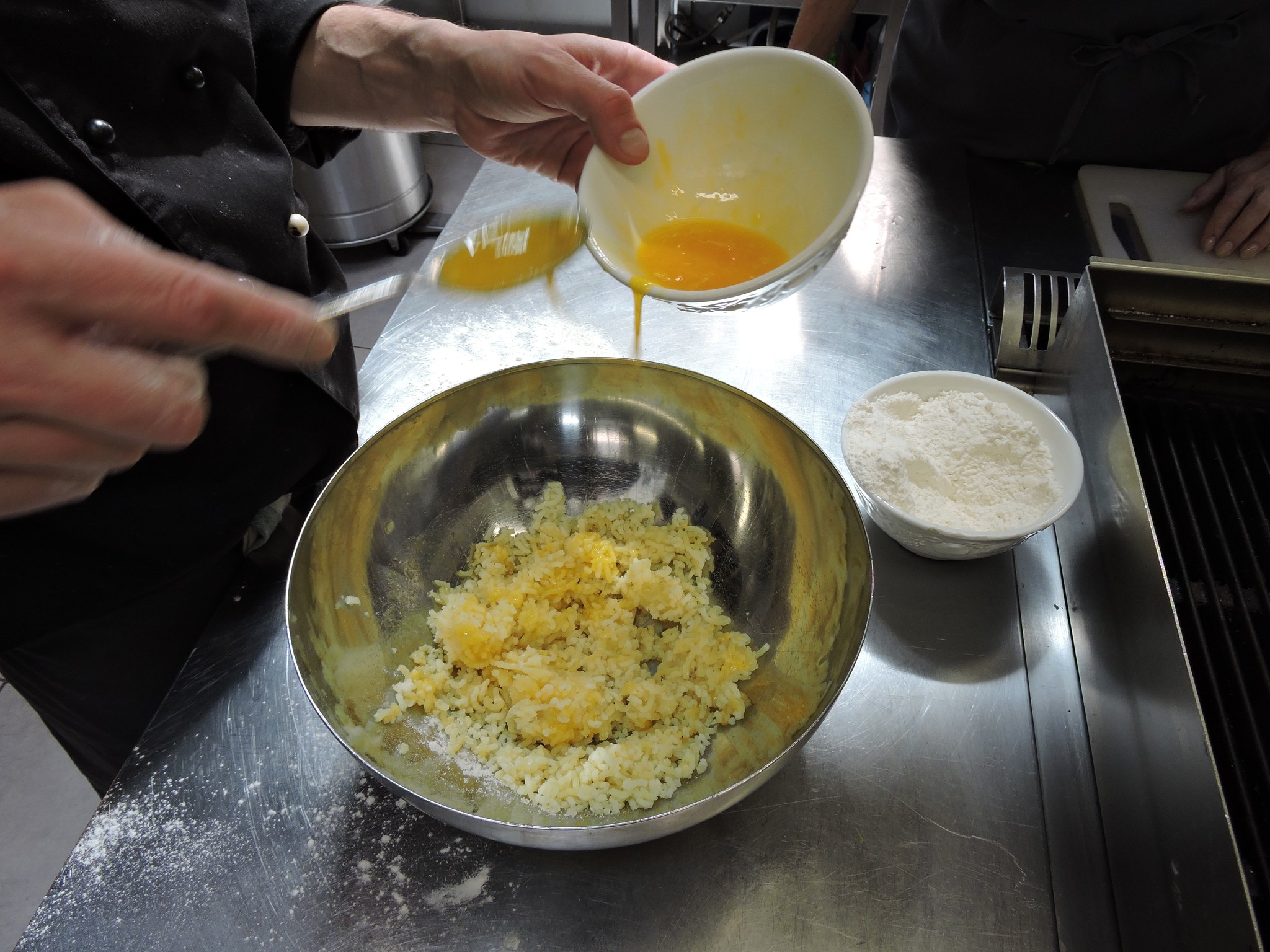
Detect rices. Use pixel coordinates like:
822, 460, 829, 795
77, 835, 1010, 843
375, 481, 770, 816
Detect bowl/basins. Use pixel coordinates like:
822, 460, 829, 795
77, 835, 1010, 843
839, 371, 1085, 560
577, 44, 876, 313
286, 352, 879, 855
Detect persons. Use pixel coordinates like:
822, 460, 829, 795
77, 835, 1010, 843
786, 0, 1270, 259
1, 1, 676, 801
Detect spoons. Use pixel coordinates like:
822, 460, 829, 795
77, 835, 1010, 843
169, 199, 588, 367
